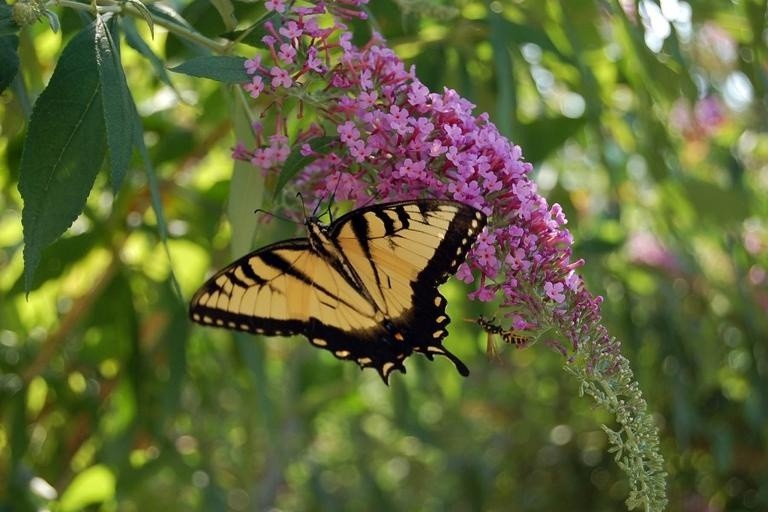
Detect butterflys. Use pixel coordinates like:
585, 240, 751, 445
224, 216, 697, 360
188, 167, 489, 390
